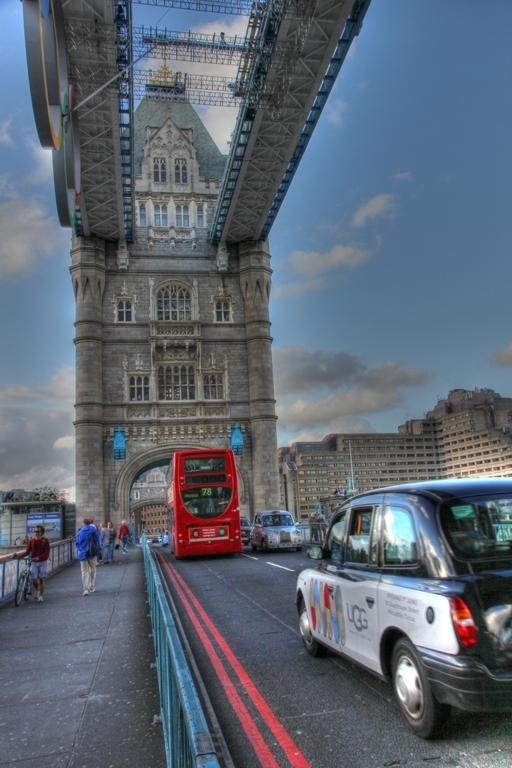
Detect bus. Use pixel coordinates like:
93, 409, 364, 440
166, 448, 242, 560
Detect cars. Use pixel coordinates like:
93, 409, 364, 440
250, 509, 304, 553
141, 533, 168, 546
295, 478, 512, 740
240, 517, 253, 546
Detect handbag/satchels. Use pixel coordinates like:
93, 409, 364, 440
87, 531, 99, 557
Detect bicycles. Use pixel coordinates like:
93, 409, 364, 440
11, 551, 44, 608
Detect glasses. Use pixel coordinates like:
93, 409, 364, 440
33, 530, 40, 533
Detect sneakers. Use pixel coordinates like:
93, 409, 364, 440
103, 560, 109, 564
82, 588, 89, 596
89, 586, 96, 592
33, 590, 44, 602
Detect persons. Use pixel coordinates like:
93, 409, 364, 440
13, 525, 52, 603
75, 517, 103, 597
89, 519, 131, 566
309, 512, 327, 541
